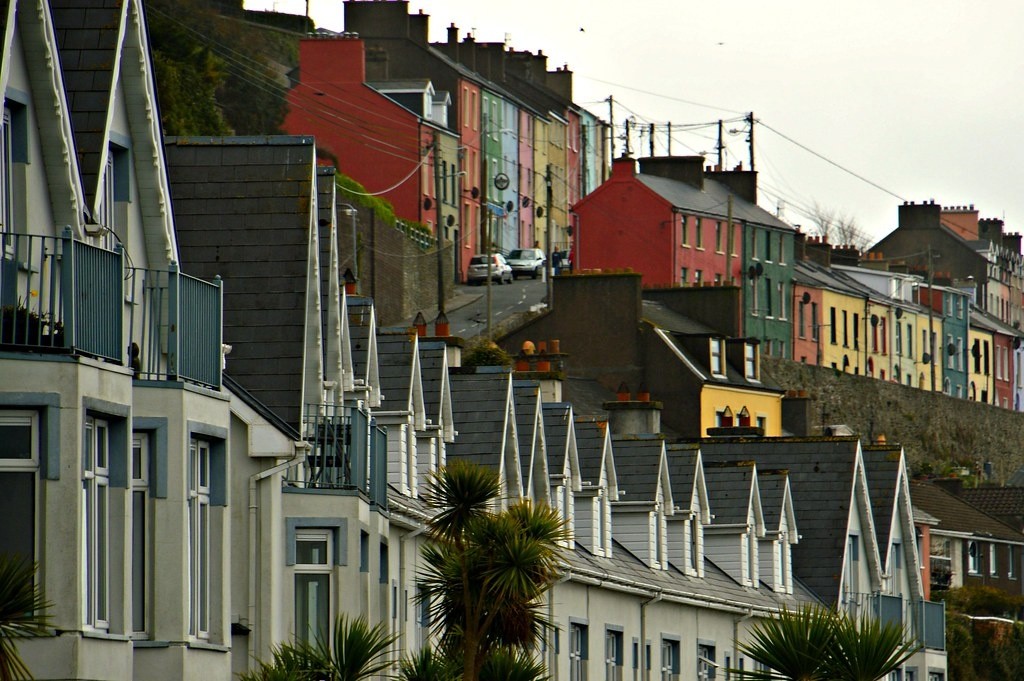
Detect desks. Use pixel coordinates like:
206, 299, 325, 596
303, 437, 345, 488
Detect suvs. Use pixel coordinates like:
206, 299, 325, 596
468, 253, 513, 284
506, 248, 547, 279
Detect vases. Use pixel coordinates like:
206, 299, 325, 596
2, 311, 44, 345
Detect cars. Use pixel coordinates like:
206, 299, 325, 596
560, 248, 571, 266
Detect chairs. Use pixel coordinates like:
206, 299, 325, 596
310, 424, 351, 487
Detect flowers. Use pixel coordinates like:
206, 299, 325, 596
2, 290, 57, 325
